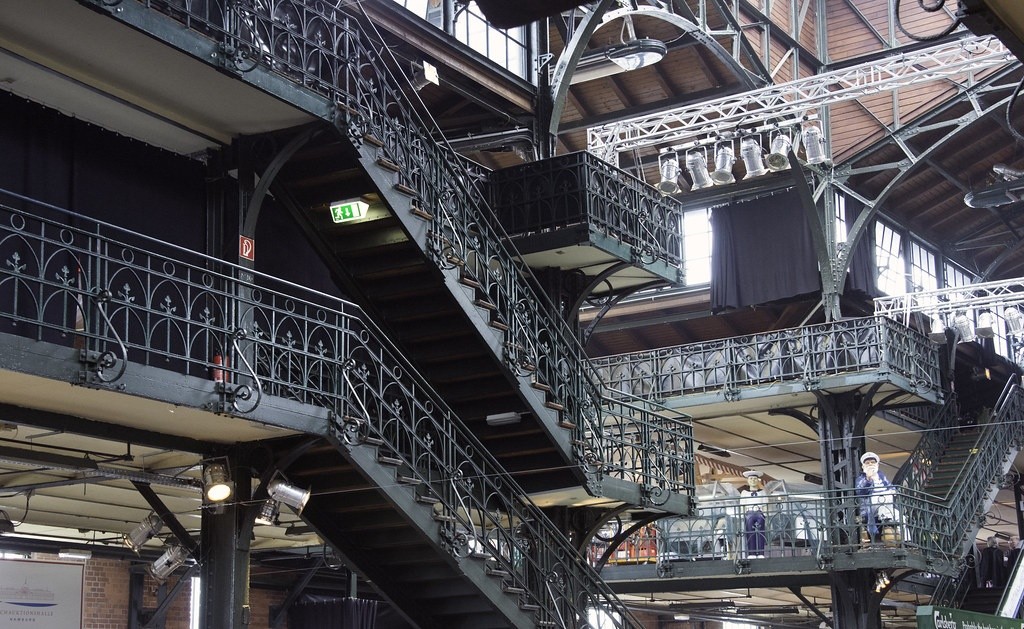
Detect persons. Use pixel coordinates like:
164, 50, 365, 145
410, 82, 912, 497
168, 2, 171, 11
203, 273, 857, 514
980, 536, 1004, 587
949, 342, 991, 432
1007, 536, 1024, 588
856, 451, 895, 550
740, 468, 769, 558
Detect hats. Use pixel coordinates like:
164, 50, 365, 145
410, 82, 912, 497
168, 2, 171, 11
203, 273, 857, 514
860, 452, 881, 464
742, 470, 763, 477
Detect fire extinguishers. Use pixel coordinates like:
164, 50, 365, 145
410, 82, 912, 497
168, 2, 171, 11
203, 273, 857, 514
214, 348, 231, 383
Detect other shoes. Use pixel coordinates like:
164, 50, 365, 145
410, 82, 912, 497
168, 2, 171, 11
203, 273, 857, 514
747, 555, 757, 559
757, 554, 765, 559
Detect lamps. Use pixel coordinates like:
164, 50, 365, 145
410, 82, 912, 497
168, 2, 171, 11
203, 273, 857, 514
739, 132, 770, 180
258, 500, 275, 522
764, 126, 793, 174
928, 313, 948, 346
75, 451, 98, 473
198, 454, 236, 503
121, 510, 162, 558
974, 309, 994, 339
953, 309, 978, 344
605, 16, 668, 72
1004, 304, 1023, 335
0, 519, 14, 537
673, 612, 691, 622
683, 141, 714, 192
59, 549, 93, 559
143, 537, 188, 587
654, 146, 683, 198
800, 121, 827, 166
284, 521, 307, 537
485, 411, 522, 426
410, 59, 440, 91
268, 468, 312, 517
708, 134, 738, 185
